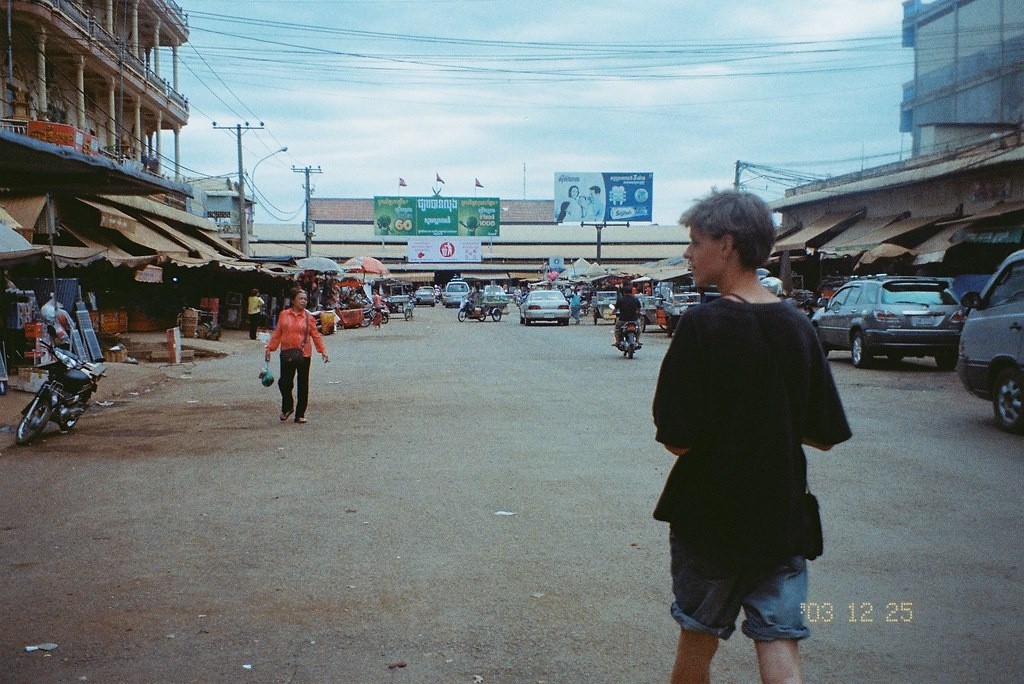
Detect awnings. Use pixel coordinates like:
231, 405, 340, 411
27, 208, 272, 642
833, 214, 943, 251
509, 272, 548, 279
773, 207, 864, 254
966, 145, 1024, 170
818, 210, 909, 257
460, 273, 510, 280
767, 148, 1014, 222
344, 272, 381, 283
907, 222, 974, 264
948, 212, 1024, 244
381, 271, 435, 281
934, 200, 1024, 226
771, 222, 802, 241
0, 182, 299, 279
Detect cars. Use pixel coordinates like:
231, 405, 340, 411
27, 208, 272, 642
811, 276, 970, 372
518, 289, 571, 326
956, 248, 1024, 434
411, 277, 529, 310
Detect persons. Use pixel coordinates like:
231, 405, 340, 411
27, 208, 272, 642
248, 288, 265, 341
653, 191, 852, 684
284, 275, 392, 329
264, 289, 331, 423
513, 283, 689, 354
556, 186, 603, 223
502, 281, 509, 293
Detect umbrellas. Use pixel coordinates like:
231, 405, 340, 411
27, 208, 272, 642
341, 255, 389, 285
853, 242, 915, 271
294, 256, 342, 272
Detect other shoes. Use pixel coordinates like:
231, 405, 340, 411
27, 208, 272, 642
636, 341, 643, 345
611, 343, 619, 346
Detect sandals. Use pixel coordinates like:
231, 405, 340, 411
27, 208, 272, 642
294, 417, 307, 423
280, 408, 294, 420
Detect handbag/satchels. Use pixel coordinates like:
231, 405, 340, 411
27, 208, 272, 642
280, 348, 303, 365
262, 361, 274, 387
804, 493, 823, 560
375, 305, 383, 311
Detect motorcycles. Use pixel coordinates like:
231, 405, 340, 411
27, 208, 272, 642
15, 325, 108, 447
607, 303, 642, 359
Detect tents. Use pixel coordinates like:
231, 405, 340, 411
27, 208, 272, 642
518, 258, 692, 294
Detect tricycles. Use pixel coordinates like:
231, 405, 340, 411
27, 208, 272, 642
458, 294, 509, 323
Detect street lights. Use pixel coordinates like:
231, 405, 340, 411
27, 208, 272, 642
250, 146, 288, 236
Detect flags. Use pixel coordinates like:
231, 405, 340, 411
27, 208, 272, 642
399, 178, 407, 185
438, 174, 445, 184
476, 179, 483, 187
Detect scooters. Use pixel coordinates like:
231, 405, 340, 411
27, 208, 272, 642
311, 301, 416, 333
